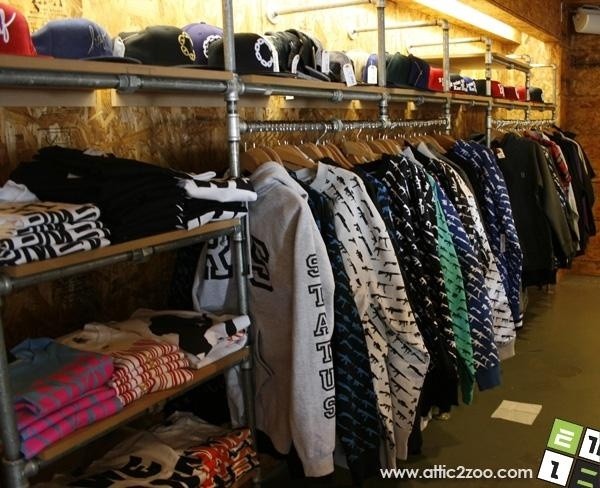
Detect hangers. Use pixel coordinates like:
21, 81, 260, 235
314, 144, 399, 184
480, 118, 558, 148
222, 118, 459, 179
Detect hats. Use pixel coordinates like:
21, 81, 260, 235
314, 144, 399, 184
0, 1, 543, 102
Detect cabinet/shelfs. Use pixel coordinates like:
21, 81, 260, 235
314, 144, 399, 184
0, 1, 564, 488
